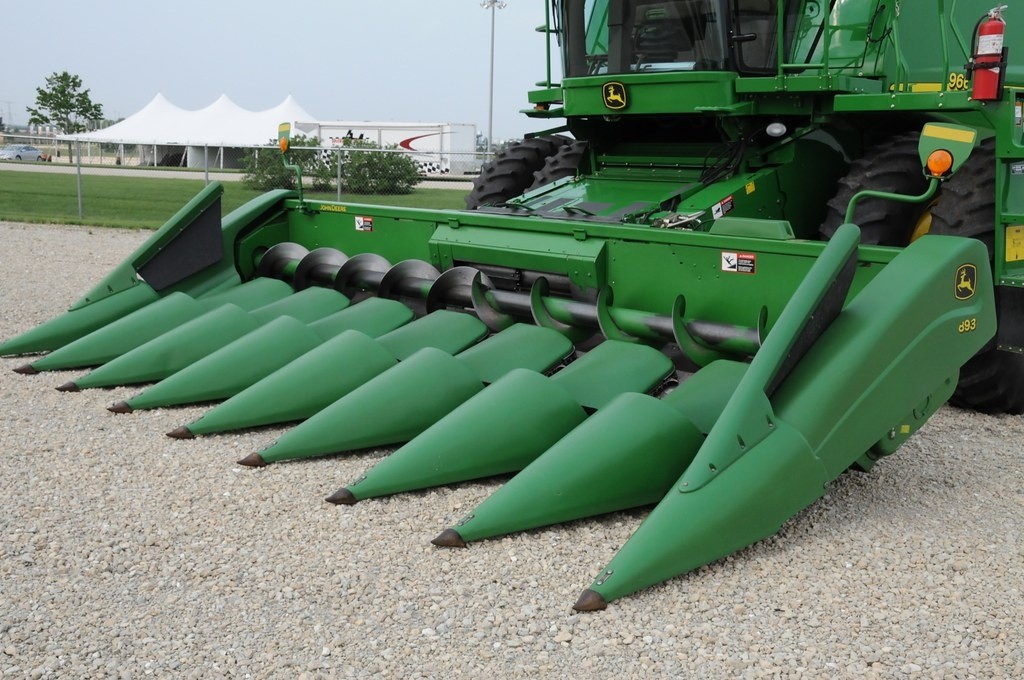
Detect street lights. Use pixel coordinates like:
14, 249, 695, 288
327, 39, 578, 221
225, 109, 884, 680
479, 1, 508, 163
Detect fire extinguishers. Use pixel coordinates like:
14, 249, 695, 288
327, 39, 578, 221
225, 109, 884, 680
964, 5, 1009, 100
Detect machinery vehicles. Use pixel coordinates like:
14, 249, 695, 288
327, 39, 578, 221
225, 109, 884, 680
0, 0, 1023, 618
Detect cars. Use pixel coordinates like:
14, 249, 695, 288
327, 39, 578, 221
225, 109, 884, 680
0, 145, 45, 163
